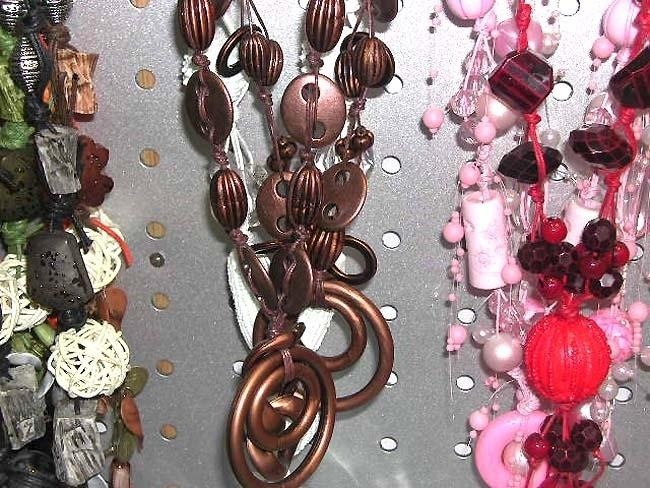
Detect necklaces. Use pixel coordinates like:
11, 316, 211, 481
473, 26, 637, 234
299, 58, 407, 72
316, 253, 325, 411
177, 0, 403, 487
421, 0, 650, 487
0, 2, 149, 487
